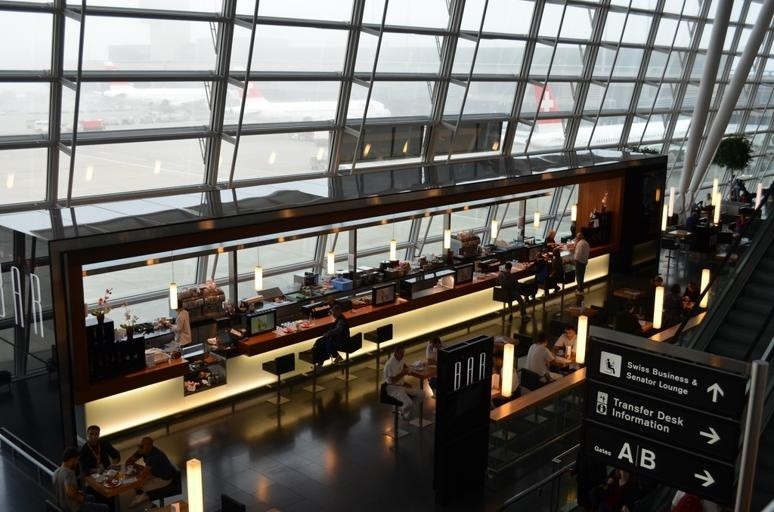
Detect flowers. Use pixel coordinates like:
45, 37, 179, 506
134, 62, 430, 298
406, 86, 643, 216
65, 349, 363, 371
90, 288, 113, 316
122, 313, 138, 328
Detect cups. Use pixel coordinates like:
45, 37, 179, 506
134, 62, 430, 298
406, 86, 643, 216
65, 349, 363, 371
127, 465, 134, 472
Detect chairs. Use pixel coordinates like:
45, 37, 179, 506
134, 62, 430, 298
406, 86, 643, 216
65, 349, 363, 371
660, 204, 763, 265
138, 467, 182, 508
262, 324, 392, 404
492, 263, 576, 326
394, 282, 708, 420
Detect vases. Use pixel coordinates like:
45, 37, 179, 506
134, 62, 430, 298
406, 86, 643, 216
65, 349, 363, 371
97, 314, 105, 325
126, 328, 133, 339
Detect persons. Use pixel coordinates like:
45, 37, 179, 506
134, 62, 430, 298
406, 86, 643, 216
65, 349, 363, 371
426, 335, 449, 398
159, 299, 192, 348
523, 249, 563, 304
614, 277, 700, 338
383, 345, 425, 420
498, 263, 531, 322
493, 351, 521, 402
52, 426, 120, 512
704, 193, 712, 206
125, 437, 176, 508
597, 470, 633, 512
573, 232, 590, 295
521, 326, 577, 390
310, 305, 349, 369
544, 230, 556, 248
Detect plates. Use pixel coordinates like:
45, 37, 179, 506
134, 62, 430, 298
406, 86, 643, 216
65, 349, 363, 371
91, 473, 102, 480
146, 346, 162, 368
104, 481, 121, 488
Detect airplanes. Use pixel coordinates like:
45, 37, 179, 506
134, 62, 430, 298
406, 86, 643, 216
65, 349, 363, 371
501, 83, 692, 149
232, 81, 392, 141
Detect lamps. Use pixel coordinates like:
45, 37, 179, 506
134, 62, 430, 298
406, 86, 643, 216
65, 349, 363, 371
186, 458, 205, 512
568, 193, 579, 223
669, 186, 675, 217
169, 259, 179, 309
387, 220, 399, 262
531, 197, 542, 230
755, 183, 763, 210
441, 225, 454, 250
713, 178, 721, 226
326, 235, 336, 276
699, 271, 713, 310
489, 216, 500, 241
251, 244, 265, 291
